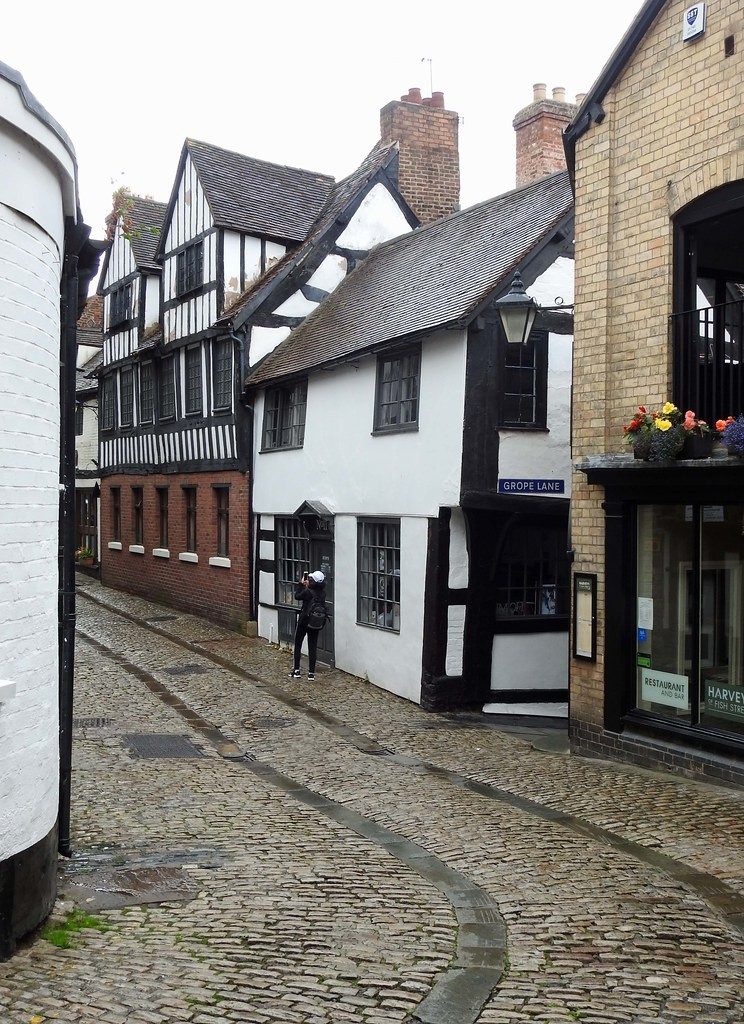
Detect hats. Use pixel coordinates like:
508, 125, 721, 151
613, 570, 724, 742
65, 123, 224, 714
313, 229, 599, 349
308, 570, 324, 583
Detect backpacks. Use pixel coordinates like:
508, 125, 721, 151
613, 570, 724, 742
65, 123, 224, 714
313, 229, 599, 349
303, 588, 327, 630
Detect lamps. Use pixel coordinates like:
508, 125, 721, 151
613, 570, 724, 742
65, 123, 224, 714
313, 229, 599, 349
493, 268, 574, 345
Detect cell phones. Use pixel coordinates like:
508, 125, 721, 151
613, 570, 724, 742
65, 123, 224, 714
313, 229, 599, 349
303, 570, 308, 581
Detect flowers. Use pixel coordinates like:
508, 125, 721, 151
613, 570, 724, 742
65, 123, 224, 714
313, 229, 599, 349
75, 546, 94, 559
621, 401, 712, 464
715, 414, 744, 457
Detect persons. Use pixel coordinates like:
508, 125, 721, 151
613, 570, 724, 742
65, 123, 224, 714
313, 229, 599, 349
288, 571, 329, 681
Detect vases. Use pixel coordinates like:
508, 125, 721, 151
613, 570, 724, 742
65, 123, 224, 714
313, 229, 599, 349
80, 557, 93, 565
634, 432, 712, 459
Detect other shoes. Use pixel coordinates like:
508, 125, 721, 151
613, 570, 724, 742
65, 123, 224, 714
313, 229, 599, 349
308, 674, 315, 681
288, 670, 301, 678
